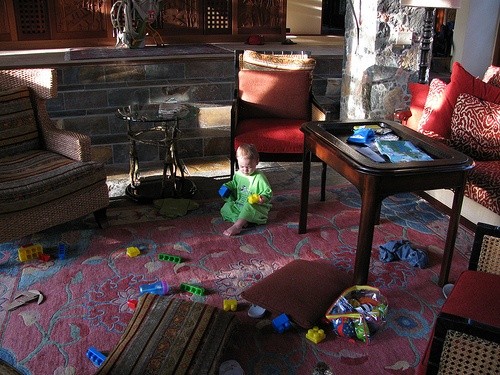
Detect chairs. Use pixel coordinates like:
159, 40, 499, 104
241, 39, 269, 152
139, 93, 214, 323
416, 221, 500, 375
0, 68, 110, 246
227, 48, 330, 203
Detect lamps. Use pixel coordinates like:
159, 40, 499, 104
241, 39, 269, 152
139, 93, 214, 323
400, 0, 462, 84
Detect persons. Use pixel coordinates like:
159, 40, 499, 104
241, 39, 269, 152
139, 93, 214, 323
220, 143, 272, 237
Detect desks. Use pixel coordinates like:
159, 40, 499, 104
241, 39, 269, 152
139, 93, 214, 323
117, 102, 200, 206
299, 117, 478, 291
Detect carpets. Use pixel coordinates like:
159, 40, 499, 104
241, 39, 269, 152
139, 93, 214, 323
0, 183, 500, 375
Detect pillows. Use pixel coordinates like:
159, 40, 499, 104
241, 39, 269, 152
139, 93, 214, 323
417, 77, 448, 142
242, 50, 317, 71
95, 291, 236, 375
422, 62, 500, 139
238, 70, 313, 121
451, 90, 500, 160
241, 259, 354, 331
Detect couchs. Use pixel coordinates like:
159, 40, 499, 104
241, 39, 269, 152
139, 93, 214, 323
405, 62, 500, 231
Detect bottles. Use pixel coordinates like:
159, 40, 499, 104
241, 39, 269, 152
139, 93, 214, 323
137, 279, 174, 296
